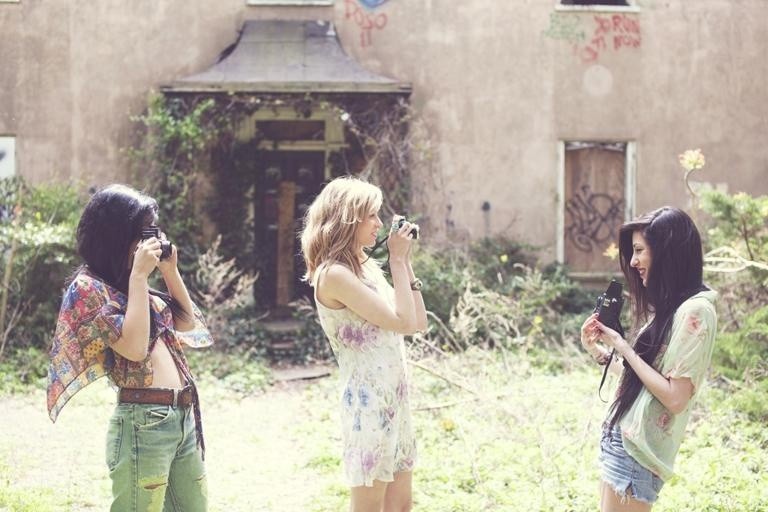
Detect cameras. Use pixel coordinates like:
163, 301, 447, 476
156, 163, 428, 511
398, 218, 419, 240
595, 280, 624, 328
141, 225, 172, 259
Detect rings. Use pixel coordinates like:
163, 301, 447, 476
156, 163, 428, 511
391, 223, 399, 233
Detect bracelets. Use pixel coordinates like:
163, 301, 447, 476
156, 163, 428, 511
590, 351, 613, 367
410, 278, 425, 290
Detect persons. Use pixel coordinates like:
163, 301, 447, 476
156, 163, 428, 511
296, 176, 431, 512
46, 180, 216, 511
579, 205, 721, 512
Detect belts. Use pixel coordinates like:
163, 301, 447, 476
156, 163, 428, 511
115, 383, 195, 410
